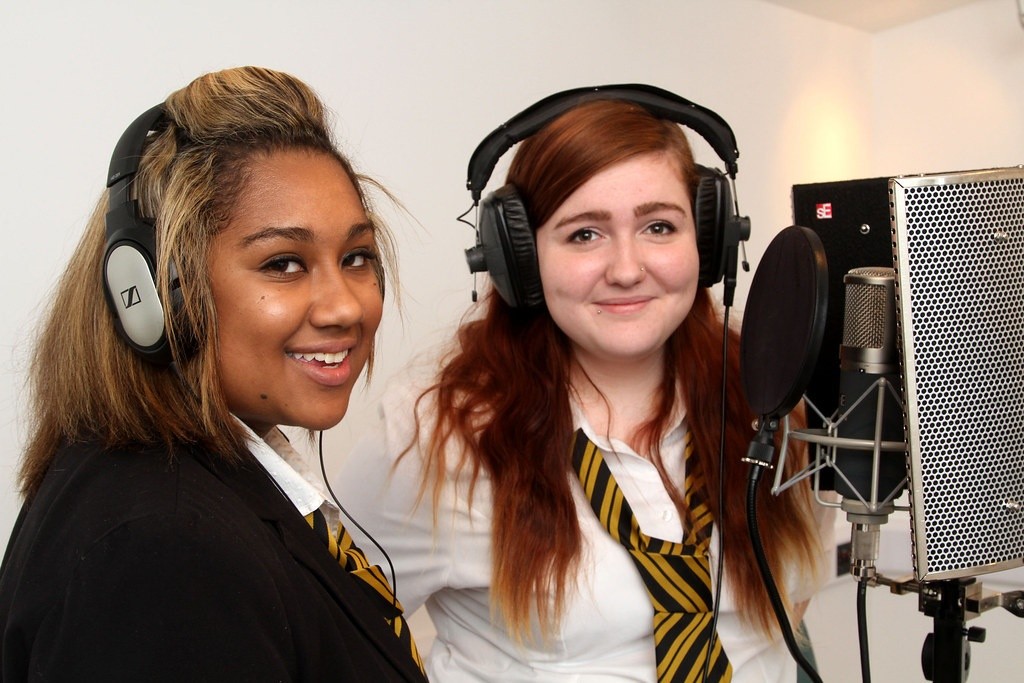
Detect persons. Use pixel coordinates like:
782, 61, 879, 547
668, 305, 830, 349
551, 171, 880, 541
1, 65, 430, 683
321, 98, 840, 683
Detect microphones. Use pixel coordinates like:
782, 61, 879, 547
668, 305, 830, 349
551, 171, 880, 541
831, 267, 907, 578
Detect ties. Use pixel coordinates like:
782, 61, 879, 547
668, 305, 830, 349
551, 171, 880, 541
572, 428, 733, 683
306, 510, 428, 682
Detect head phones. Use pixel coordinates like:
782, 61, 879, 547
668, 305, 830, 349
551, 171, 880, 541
99, 101, 198, 365
458, 84, 751, 314
